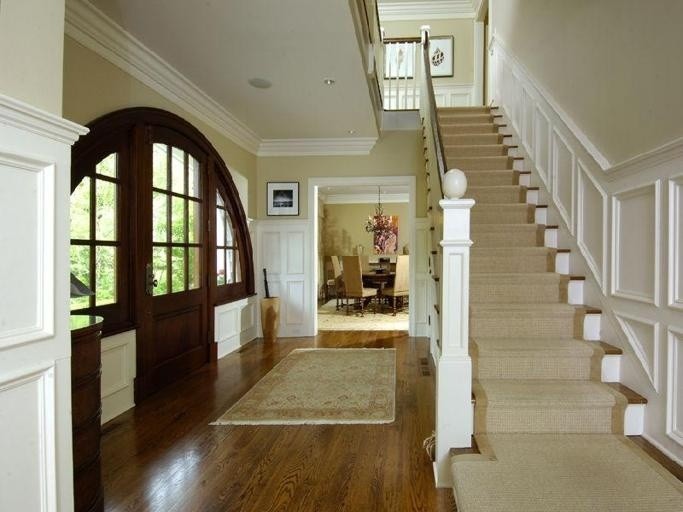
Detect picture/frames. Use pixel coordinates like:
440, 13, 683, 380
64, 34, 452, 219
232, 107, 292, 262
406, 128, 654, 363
265, 181, 299, 217
384, 35, 455, 81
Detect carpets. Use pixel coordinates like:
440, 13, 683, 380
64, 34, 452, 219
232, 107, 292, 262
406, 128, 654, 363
317, 298, 408, 331
207, 346, 397, 426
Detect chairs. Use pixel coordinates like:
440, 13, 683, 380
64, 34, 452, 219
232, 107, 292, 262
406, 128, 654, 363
324, 255, 408, 318
